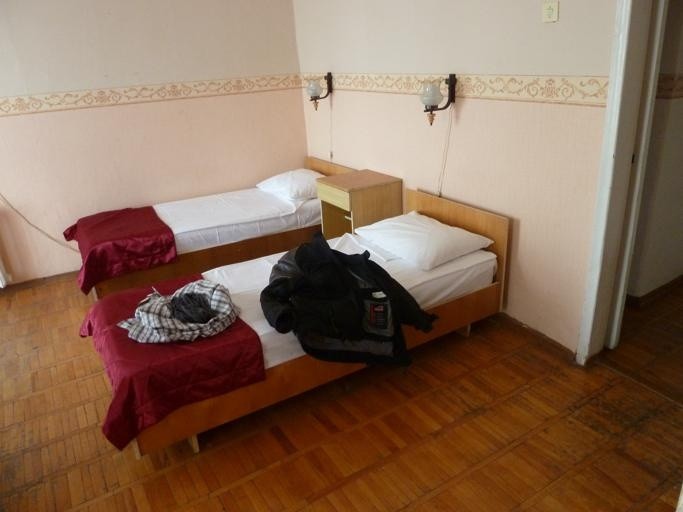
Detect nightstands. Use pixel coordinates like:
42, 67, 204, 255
315, 169, 402, 241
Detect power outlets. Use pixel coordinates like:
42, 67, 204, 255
541, 3, 559, 22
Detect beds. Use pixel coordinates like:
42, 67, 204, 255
79, 189, 510, 460
63, 156, 358, 304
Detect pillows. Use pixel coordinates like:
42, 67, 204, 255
256, 168, 326, 200
355, 209, 496, 273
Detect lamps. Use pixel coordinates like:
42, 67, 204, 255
303, 72, 332, 111
420, 73, 456, 125
